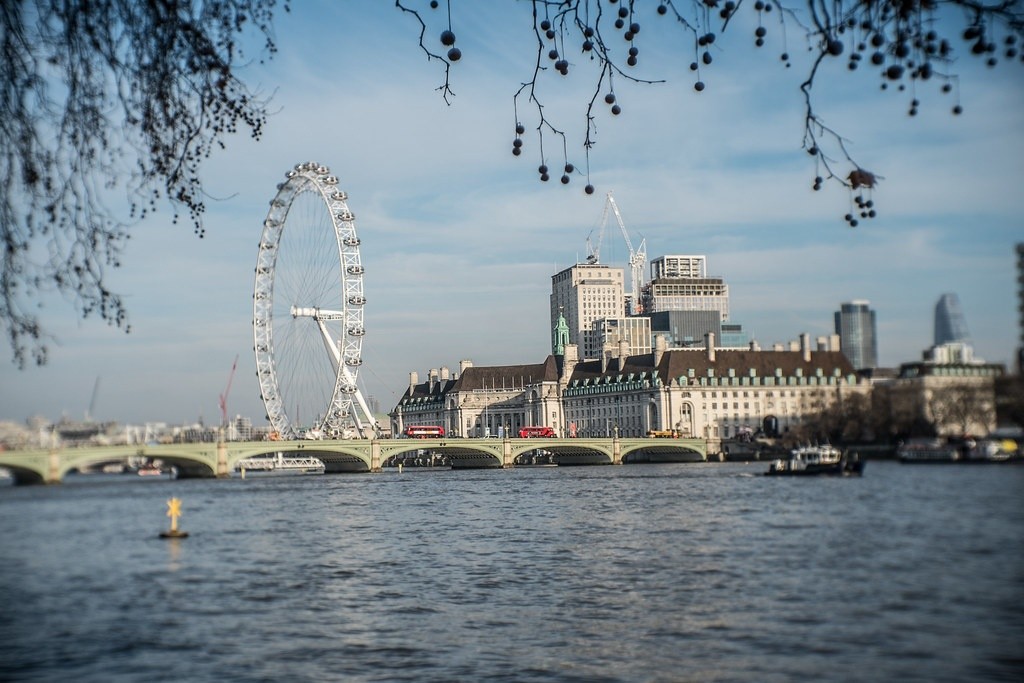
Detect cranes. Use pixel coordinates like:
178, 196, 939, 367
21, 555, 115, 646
219, 353, 239, 425
586, 191, 647, 315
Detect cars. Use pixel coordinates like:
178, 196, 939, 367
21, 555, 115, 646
298, 430, 315, 441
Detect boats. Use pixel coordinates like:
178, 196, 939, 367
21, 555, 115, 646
763, 439, 866, 475
900, 436, 1016, 462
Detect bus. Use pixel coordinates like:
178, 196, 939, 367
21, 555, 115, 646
404, 425, 444, 438
517, 427, 554, 438
